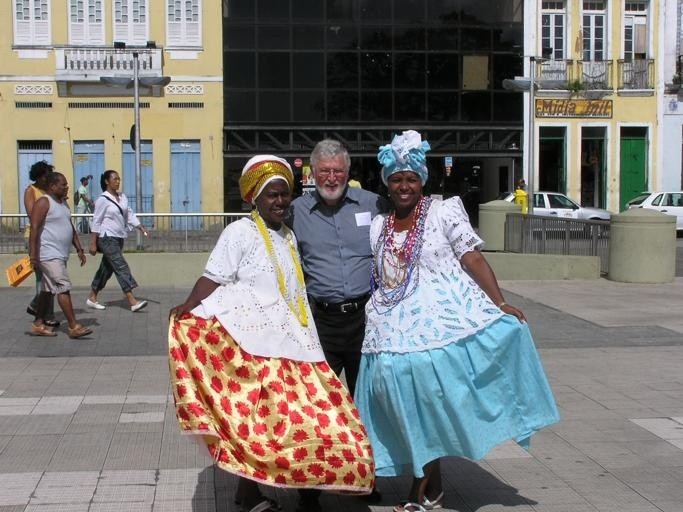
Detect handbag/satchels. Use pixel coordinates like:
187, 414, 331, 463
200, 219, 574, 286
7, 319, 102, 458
26, 294, 54, 319
7, 256, 34, 287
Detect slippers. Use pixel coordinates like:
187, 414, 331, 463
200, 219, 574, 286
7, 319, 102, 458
233, 492, 282, 511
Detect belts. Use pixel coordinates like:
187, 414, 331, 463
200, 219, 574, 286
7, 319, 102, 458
306, 295, 374, 315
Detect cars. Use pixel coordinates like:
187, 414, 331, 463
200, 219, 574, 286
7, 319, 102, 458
485, 187, 615, 254
620, 184, 683, 240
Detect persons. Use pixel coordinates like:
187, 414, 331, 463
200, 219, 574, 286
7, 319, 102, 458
75, 175, 95, 233
517, 178, 527, 193
27, 172, 93, 339
168, 154, 375, 512
86, 170, 148, 312
24, 160, 68, 327
286, 140, 391, 511
354, 129, 559, 512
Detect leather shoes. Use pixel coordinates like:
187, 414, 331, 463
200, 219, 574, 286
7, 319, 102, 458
361, 492, 384, 504
44, 319, 60, 326
295, 500, 324, 512
87, 299, 106, 310
31, 323, 58, 337
68, 324, 93, 339
130, 300, 148, 312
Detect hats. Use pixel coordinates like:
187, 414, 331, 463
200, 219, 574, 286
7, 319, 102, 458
377, 129, 432, 188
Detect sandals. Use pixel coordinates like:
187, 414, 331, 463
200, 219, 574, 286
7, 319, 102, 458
393, 491, 444, 510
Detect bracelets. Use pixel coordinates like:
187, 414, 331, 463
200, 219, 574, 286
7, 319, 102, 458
498, 302, 508, 310
77, 248, 83, 253
